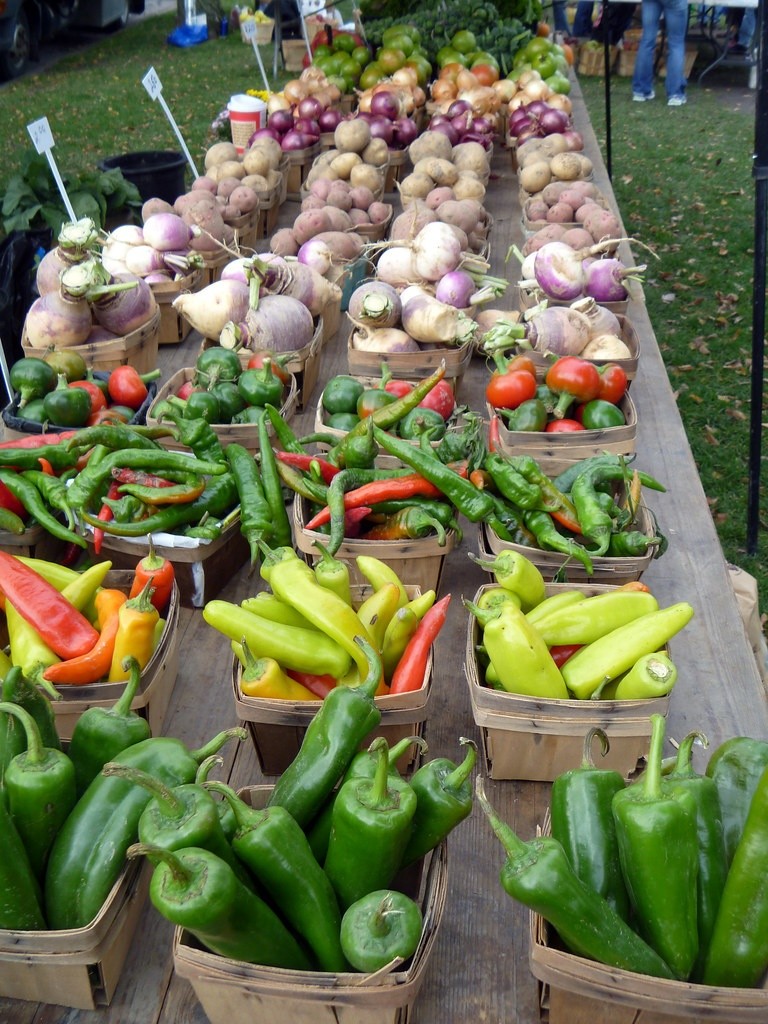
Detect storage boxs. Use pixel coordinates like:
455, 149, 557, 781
0, 133, 767, 1023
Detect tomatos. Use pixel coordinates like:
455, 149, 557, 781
313, 25, 572, 95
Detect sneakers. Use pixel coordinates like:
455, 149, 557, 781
632, 90, 656, 101
668, 96, 686, 106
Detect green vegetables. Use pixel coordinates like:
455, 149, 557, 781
2, 147, 142, 241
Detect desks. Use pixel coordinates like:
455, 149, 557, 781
0, 61, 768, 1023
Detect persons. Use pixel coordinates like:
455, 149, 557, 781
553, 0, 594, 38
632, 0, 688, 105
691, 4, 757, 55
255, 0, 302, 38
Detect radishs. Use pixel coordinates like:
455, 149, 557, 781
23, 214, 645, 361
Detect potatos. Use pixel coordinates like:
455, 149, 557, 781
142, 119, 624, 258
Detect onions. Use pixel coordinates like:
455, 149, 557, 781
252, 68, 584, 151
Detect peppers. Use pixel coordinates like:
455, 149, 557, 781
0, 347, 768, 991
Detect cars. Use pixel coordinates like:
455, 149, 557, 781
0, 0, 147, 80
